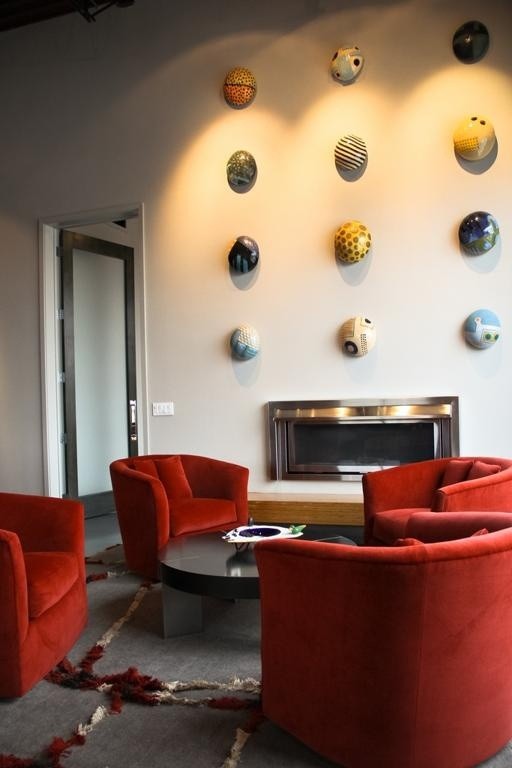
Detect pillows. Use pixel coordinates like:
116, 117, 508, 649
134, 454, 193, 501
465, 527, 493, 535
467, 460, 502, 483
440, 460, 473, 491
390, 536, 427, 547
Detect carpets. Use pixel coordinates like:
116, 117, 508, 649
0, 542, 512, 768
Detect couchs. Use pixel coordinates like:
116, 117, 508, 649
106, 452, 251, 583
252, 510, 512, 768
362, 457, 512, 546
1, 490, 90, 699
